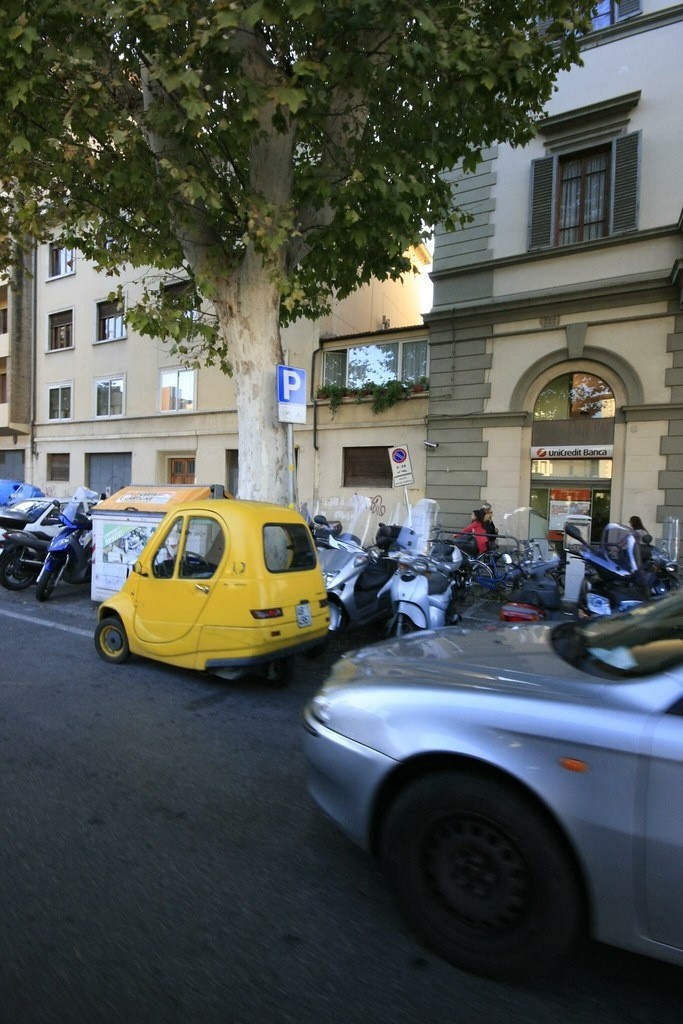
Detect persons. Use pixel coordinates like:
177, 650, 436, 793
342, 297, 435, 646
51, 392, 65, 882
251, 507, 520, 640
628, 516, 650, 546
451, 503, 498, 555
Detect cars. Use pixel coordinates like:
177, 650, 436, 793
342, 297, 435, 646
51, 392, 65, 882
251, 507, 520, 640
0, 496, 102, 554
296, 585, 683, 987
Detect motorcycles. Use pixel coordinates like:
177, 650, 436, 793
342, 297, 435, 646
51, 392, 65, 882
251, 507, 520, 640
304, 514, 683, 656
36, 486, 109, 603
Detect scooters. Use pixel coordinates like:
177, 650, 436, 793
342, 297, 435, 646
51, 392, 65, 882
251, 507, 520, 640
0, 507, 52, 589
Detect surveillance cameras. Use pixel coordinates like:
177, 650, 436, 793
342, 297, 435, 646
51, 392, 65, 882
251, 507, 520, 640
423, 440, 438, 447
